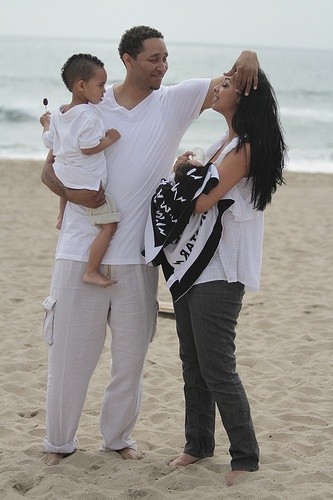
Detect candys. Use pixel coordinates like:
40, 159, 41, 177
42, 98, 48, 113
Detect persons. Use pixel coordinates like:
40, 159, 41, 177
40, 55, 120, 287
41, 26, 259, 468
141, 66, 289, 487
